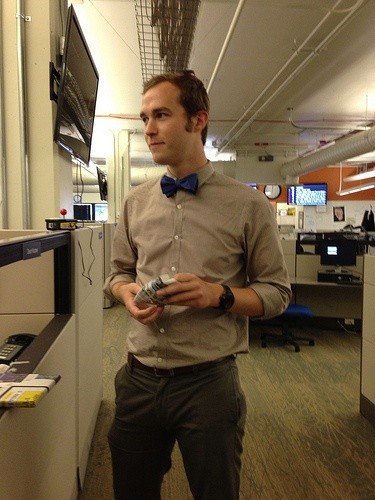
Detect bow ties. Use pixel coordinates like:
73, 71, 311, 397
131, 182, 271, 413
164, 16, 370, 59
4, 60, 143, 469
160, 174, 197, 199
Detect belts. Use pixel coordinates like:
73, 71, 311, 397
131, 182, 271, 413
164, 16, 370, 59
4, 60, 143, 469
130, 355, 234, 376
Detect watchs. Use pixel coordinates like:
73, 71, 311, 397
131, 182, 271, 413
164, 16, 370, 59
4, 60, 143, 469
212, 284, 235, 311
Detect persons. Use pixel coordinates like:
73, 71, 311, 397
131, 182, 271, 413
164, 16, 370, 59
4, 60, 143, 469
103, 71, 293, 500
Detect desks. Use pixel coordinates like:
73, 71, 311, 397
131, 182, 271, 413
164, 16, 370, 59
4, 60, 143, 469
233, 202, 375, 354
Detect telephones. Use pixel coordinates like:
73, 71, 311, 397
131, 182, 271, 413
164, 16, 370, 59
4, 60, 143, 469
0, 333, 37, 365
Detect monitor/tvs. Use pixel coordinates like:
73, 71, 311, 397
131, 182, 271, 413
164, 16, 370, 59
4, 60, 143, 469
53, 3, 100, 166
287, 183, 327, 206
318, 240, 356, 273
73, 202, 108, 222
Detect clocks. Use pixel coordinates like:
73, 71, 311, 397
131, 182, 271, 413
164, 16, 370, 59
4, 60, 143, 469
263, 184, 281, 200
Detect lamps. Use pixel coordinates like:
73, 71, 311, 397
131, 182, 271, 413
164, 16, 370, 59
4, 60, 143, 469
336, 155, 375, 197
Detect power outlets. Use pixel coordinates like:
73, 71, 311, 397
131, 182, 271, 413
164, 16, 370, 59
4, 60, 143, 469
344, 318, 354, 325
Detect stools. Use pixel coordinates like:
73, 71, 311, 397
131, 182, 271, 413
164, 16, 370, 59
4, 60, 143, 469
257, 304, 316, 353
